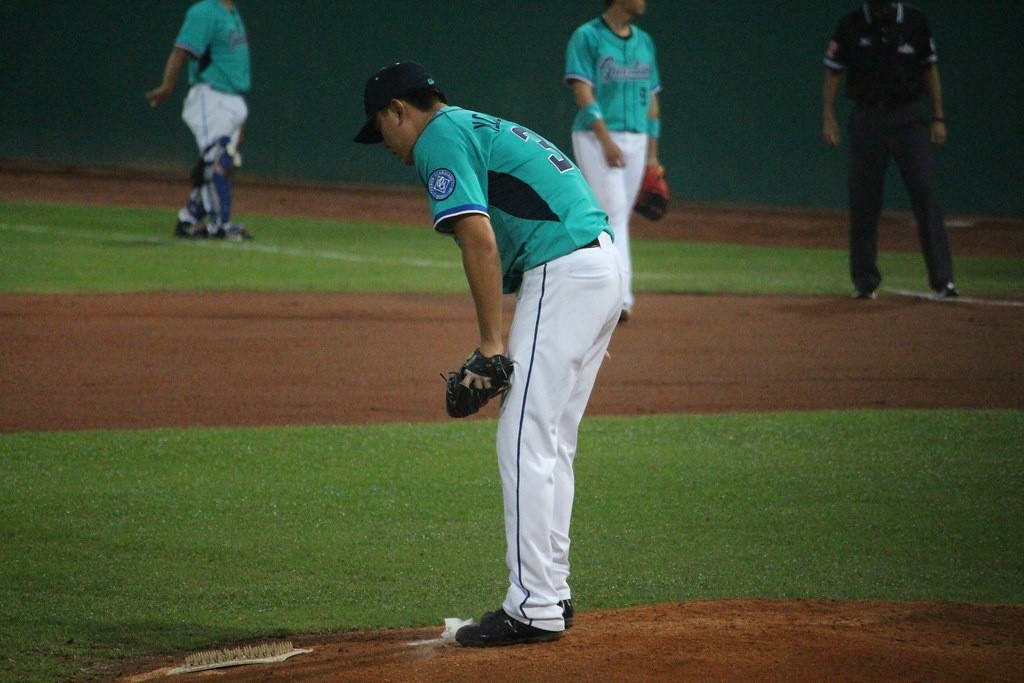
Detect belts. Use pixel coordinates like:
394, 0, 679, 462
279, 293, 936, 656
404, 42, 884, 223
579, 238, 600, 248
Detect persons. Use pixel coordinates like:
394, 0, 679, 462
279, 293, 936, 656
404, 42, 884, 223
562, 0, 669, 321
819, 0, 964, 300
351, 60, 628, 649
145, 0, 257, 242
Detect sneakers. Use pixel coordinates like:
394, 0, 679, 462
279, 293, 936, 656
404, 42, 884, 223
854, 290, 876, 300
934, 281, 959, 299
455, 613, 563, 648
480, 599, 574, 628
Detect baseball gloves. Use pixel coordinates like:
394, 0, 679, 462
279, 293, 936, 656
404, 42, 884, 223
445, 348, 514, 419
634, 165, 670, 220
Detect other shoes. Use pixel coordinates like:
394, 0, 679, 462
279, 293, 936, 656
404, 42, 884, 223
210, 225, 250, 241
617, 306, 629, 323
176, 222, 209, 238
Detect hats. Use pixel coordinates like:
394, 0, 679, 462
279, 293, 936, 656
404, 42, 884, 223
352, 61, 443, 145
